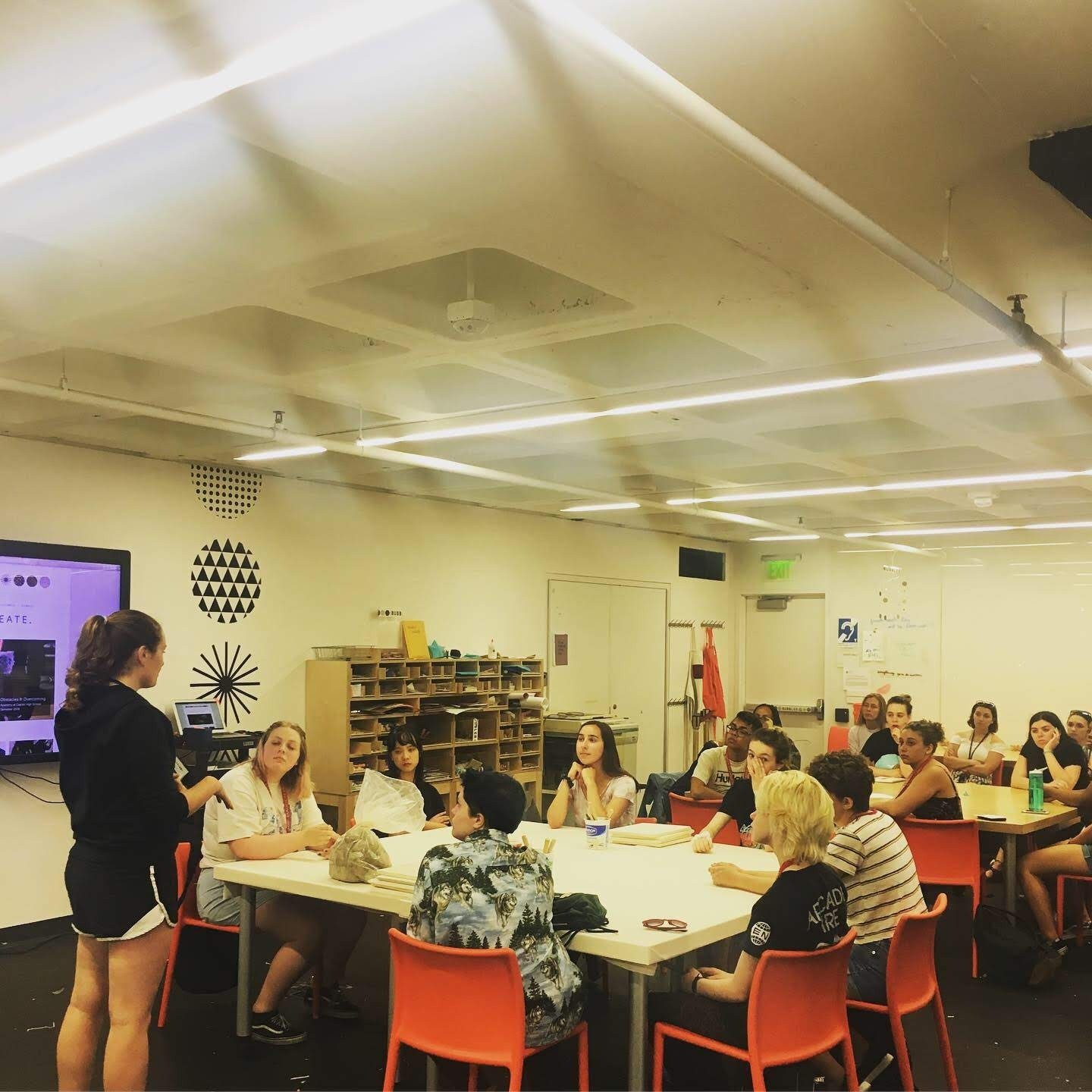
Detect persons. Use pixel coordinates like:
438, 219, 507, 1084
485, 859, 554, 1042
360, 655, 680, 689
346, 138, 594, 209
690, 711, 763, 801
872, 719, 963, 820
692, 727, 790, 852
197, 721, 362, 1045
708, 749, 929, 1083
370, 725, 452, 838
1016, 710, 1092, 957
681, 769, 871, 1092
986, 711, 1089, 882
943, 700, 1007, 786
546, 720, 640, 829
848, 693, 913, 778
55, 610, 234, 1092
753, 704, 801, 771
406, 767, 585, 1049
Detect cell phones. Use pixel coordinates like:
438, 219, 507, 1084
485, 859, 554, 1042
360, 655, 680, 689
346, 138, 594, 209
1023, 810, 1050, 814
977, 813, 1007, 821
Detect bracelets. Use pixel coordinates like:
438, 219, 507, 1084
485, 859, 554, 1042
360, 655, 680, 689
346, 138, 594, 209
692, 974, 703, 992
1041, 751, 1054, 754
558, 774, 574, 789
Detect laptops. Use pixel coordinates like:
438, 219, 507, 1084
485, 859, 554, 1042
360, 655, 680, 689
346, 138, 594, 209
172, 699, 252, 739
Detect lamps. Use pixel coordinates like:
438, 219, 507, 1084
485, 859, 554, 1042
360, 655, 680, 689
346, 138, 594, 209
231, 318, 1090, 542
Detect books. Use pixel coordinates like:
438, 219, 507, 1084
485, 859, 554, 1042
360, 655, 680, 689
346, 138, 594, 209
609, 822, 695, 848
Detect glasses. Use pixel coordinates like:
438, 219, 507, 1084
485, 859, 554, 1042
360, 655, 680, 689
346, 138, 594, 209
642, 919, 688, 932
726, 725, 752, 737
1070, 710, 1091, 718
976, 700, 995, 708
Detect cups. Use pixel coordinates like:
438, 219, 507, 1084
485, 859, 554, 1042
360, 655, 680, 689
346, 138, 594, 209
584, 816, 610, 851
541, 848, 553, 863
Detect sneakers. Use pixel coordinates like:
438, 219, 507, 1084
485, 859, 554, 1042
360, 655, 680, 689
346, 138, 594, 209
856, 1043, 894, 1092
304, 981, 361, 1019
250, 1011, 308, 1046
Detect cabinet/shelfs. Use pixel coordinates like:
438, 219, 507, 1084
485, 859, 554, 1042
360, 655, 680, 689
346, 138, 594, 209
305, 659, 544, 835
174, 736, 261, 783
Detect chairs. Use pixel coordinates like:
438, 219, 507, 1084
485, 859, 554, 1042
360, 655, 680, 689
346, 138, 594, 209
382, 928, 590, 1092
157, 816, 322, 1029
651, 725, 1092, 1091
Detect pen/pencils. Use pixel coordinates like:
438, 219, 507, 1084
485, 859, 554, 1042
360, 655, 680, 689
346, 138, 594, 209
586, 814, 591, 820
592, 814, 595, 820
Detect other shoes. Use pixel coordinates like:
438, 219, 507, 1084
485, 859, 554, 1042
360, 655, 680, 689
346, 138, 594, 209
985, 867, 1003, 882
1062, 920, 1092, 940
1047, 936, 1068, 956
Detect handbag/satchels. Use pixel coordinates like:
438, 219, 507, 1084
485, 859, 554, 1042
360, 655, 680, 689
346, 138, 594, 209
973, 904, 1056, 991
551, 892, 618, 947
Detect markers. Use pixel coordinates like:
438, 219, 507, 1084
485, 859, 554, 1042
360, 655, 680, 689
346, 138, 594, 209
544, 838, 550, 853
605, 809, 614, 820
548, 838, 557, 853
522, 835, 529, 847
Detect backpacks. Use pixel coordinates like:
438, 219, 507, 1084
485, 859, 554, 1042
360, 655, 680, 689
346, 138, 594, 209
174, 839, 239, 994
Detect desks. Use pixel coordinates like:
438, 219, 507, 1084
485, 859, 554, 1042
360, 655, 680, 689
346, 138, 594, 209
215, 822, 779, 1091
933, 743, 1018, 787
869, 774, 1083, 924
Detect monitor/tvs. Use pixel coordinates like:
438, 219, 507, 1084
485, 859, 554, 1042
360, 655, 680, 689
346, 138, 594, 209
0, 540, 130, 765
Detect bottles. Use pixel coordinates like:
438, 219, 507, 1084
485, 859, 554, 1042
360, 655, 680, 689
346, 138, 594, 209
1028, 769, 1044, 812
488, 639, 496, 660
